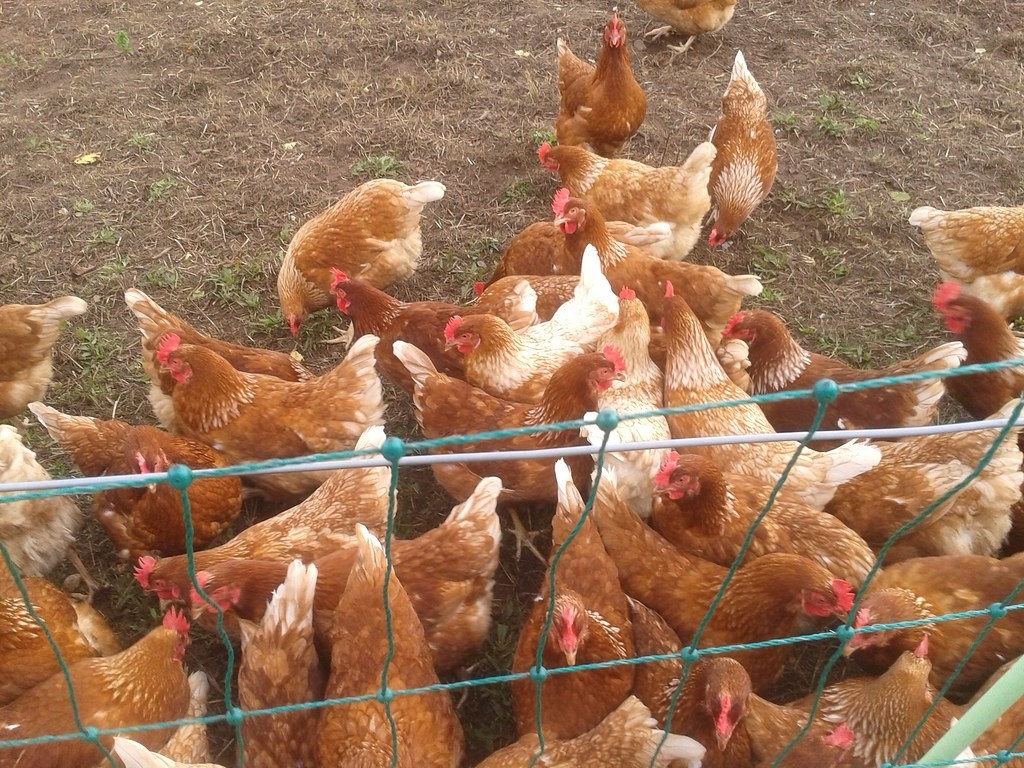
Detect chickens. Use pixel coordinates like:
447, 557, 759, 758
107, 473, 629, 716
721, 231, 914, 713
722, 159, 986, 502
0, 0, 1024, 768
